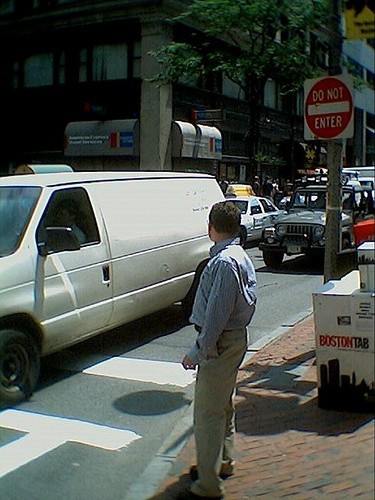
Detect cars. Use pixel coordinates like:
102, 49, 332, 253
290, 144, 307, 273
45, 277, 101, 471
342, 167, 375, 206
226, 196, 288, 248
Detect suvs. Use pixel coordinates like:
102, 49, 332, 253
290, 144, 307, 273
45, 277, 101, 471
259, 185, 375, 269
279, 169, 361, 211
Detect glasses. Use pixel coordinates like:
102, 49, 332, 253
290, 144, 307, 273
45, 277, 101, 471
206, 219, 211, 226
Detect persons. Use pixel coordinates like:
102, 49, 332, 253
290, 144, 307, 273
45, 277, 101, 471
252, 175, 296, 207
218, 174, 230, 195
172, 202, 258, 500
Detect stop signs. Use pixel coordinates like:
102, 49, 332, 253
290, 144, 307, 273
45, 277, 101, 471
303, 74, 354, 140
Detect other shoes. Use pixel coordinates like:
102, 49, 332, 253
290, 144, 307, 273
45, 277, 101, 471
190, 465, 228, 481
176, 487, 224, 500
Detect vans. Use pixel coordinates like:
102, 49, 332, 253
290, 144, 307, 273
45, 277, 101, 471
0, 163, 227, 403
